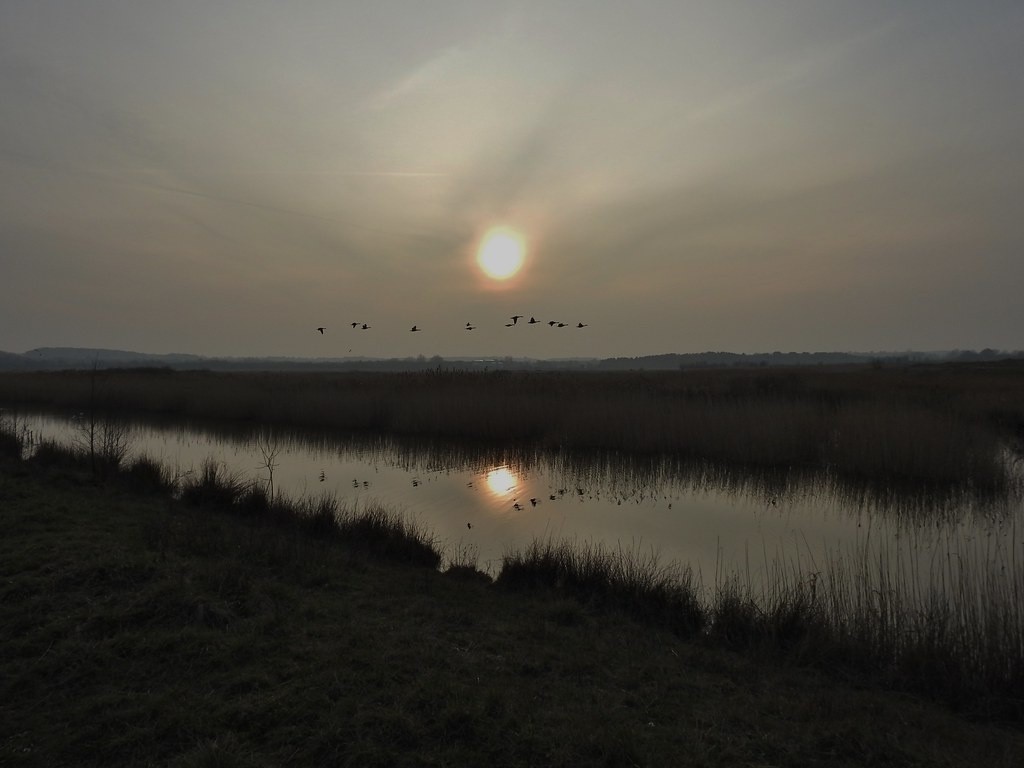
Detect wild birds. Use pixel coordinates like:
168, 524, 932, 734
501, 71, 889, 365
361, 324, 372, 329
547, 321, 559, 326
467, 322, 471, 326
576, 323, 588, 328
557, 323, 568, 327
505, 315, 524, 327
466, 327, 476, 330
317, 328, 327, 334
351, 322, 360, 328
410, 325, 421, 331
